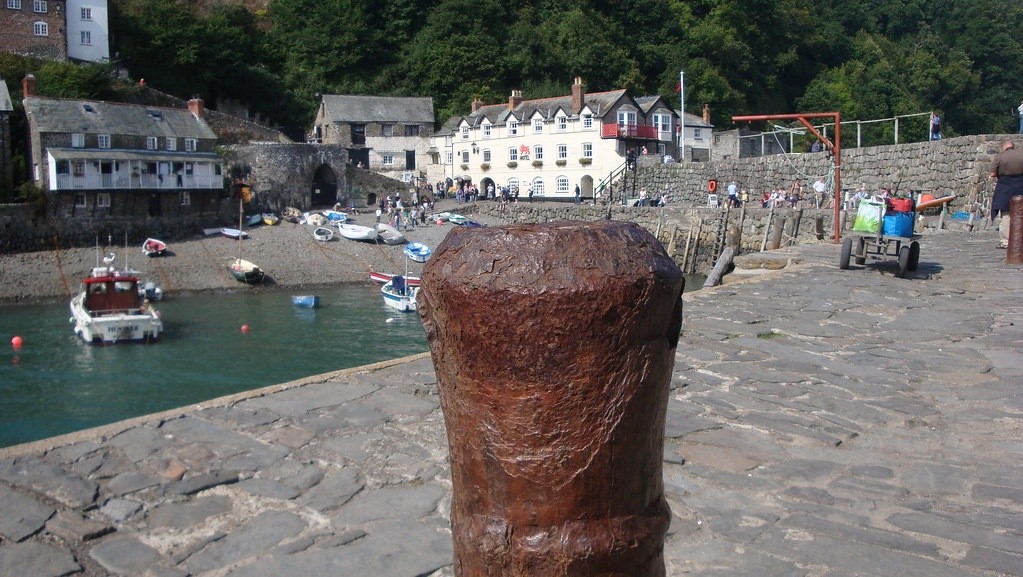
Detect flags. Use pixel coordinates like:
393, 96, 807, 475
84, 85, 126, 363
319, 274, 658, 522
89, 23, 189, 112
674, 81, 680, 93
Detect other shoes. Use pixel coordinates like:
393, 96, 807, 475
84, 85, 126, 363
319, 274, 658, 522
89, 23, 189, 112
996, 243, 1008, 249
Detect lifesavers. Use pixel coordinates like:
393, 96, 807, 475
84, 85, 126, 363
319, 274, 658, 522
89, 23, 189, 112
709, 181, 716, 191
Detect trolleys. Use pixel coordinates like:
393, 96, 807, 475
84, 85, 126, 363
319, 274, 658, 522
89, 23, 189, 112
840, 201, 919, 278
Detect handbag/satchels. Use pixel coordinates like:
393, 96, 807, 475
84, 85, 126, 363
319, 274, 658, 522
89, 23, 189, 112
853, 195, 887, 234
890, 197, 911, 211
882, 211, 914, 237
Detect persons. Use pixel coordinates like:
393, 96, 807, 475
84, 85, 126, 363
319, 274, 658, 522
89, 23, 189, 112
1017, 101, 1023, 134
811, 140, 820, 152
626, 145, 647, 170
638, 187, 667, 207
728, 176, 914, 209
177, 168, 182, 187
990, 136, 1022, 249
931, 113, 941, 141
333, 177, 533, 232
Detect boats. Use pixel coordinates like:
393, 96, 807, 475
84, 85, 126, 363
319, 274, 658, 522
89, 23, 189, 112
247, 213, 261, 227
449, 214, 465, 225
314, 226, 333, 241
338, 222, 378, 239
221, 227, 249, 239
142, 237, 168, 257
433, 212, 451, 222
405, 243, 431, 263
371, 270, 420, 287
226, 198, 265, 283
916, 194, 956, 211
308, 213, 325, 226
329, 215, 347, 226
281, 205, 302, 222
322, 209, 349, 218
69, 231, 164, 345
262, 213, 279, 225
291, 295, 320, 308
371, 223, 405, 245
380, 257, 420, 313
